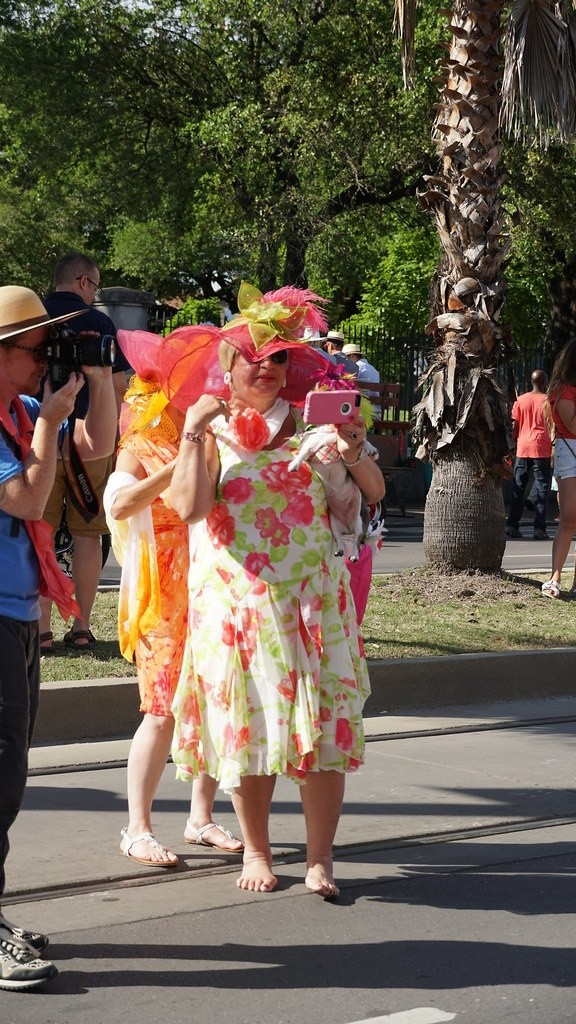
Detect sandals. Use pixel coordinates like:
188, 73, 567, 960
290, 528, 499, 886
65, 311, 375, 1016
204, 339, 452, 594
542, 580, 561, 599
63, 624, 96, 650
40, 630, 55, 653
183, 817, 245, 853
118, 826, 179, 866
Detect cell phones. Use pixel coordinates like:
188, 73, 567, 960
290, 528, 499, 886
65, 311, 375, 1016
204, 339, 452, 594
303, 390, 361, 424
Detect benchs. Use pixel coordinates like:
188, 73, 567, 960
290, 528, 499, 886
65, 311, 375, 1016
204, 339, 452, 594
355, 381, 416, 431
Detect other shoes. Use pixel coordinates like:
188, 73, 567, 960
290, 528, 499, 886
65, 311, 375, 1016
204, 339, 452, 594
555, 514, 561, 521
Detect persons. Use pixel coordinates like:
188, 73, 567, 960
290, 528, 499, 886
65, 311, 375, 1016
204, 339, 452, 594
300, 328, 381, 420
542, 341, 576, 599
160, 283, 384, 897
505, 370, 560, 539
40, 252, 131, 653
103, 335, 246, 865
0, 284, 119, 989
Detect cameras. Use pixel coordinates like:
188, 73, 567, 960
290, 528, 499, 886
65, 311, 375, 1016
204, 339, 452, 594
43, 323, 118, 389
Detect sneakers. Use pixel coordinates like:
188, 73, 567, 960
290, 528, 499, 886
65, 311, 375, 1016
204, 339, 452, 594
505, 526, 524, 538
531, 530, 549, 540
0, 946, 58, 990
0, 914, 49, 951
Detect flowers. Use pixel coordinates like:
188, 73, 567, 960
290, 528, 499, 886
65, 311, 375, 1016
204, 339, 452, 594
304, 359, 356, 391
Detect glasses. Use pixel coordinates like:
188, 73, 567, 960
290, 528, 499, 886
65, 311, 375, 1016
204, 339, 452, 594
4, 340, 48, 363
235, 348, 287, 366
74, 276, 102, 297
323, 341, 330, 349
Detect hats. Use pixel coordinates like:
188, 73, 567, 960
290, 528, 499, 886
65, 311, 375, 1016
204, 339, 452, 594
299, 327, 328, 342
322, 331, 345, 343
0, 285, 89, 345
342, 344, 367, 356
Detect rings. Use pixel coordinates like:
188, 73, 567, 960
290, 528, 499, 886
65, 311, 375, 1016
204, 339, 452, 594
222, 400, 228, 410
351, 432, 358, 440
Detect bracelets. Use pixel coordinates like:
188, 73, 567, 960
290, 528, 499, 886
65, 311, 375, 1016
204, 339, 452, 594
181, 432, 205, 444
344, 447, 365, 467
